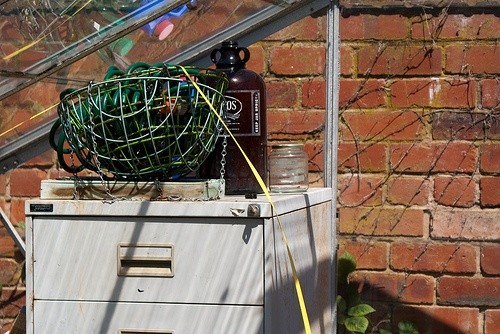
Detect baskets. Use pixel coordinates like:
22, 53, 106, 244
57, 76, 233, 180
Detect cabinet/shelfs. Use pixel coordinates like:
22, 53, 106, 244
24, 185, 335, 334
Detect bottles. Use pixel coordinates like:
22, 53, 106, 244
207, 40, 268, 195
268, 144, 309, 193
